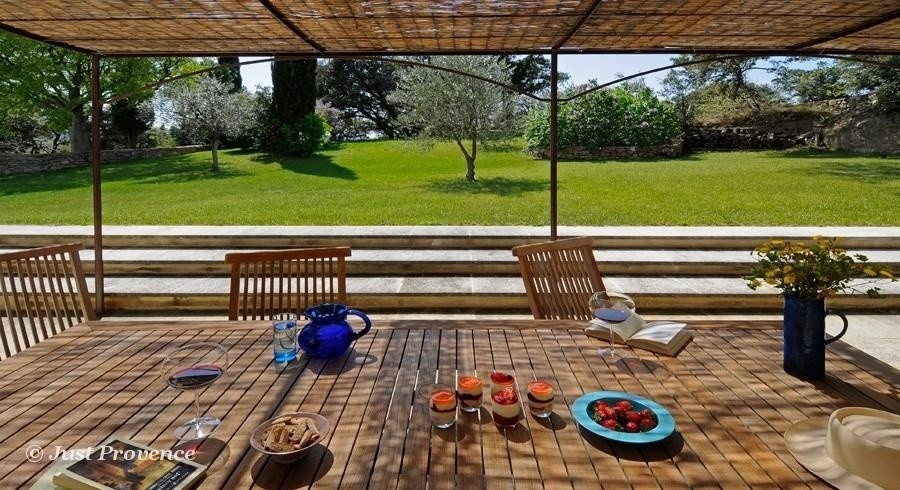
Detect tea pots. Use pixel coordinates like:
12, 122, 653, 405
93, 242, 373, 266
297, 304, 372, 359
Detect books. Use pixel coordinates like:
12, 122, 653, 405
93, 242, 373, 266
584, 302, 693, 358
53, 437, 207, 490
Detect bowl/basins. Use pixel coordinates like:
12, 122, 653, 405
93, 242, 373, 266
250, 413, 330, 461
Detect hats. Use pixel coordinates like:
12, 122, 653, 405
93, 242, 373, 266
784, 407, 900, 490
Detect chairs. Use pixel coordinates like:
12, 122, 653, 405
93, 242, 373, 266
0, 244, 94, 357
512, 236, 608, 323
225, 244, 353, 329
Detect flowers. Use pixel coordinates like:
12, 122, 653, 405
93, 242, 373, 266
742, 234, 899, 298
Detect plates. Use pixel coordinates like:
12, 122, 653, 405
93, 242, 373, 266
573, 391, 676, 444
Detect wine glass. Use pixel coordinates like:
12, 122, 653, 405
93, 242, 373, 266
587, 289, 636, 361
160, 342, 228, 442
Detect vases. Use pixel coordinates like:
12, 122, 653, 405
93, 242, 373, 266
783, 288, 846, 367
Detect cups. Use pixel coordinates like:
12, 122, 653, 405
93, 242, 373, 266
491, 386, 522, 427
458, 377, 481, 412
428, 384, 457, 429
780, 290, 848, 381
527, 382, 554, 418
488, 369, 522, 405
273, 313, 296, 363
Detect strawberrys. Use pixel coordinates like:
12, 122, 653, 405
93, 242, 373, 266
593, 400, 656, 432
